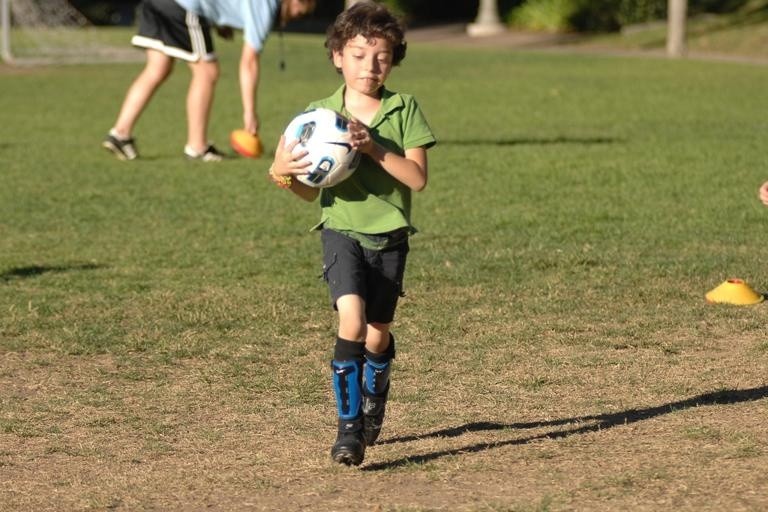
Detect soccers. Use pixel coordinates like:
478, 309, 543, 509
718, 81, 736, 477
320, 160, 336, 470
284, 108, 361, 187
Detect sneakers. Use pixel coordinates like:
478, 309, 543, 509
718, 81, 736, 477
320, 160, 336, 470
331, 407, 366, 466
102, 128, 140, 161
184, 143, 226, 162
362, 379, 391, 446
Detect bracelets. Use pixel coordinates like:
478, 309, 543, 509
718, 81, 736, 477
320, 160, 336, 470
267, 167, 294, 191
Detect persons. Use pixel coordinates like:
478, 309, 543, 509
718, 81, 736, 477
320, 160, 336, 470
102, 0, 316, 167
269, 1, 437, 466
758, 181, 767, 207
